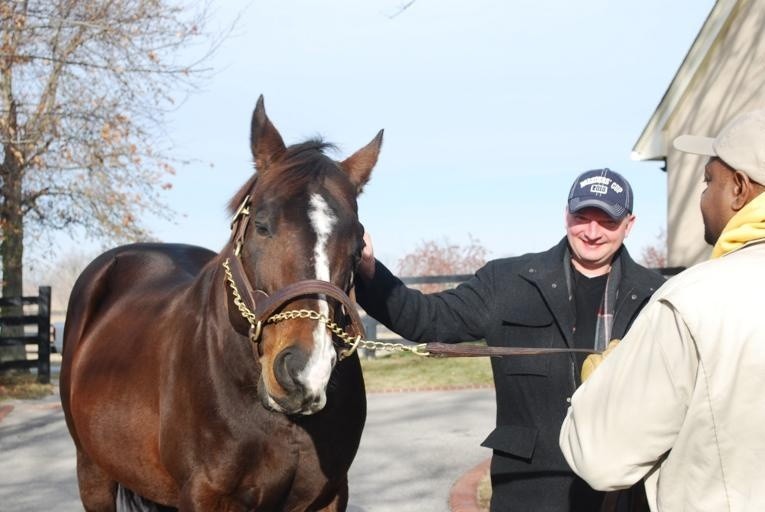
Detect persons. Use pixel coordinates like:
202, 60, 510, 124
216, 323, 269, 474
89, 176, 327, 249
352, 168, 676, 509
556, 108, 765, 510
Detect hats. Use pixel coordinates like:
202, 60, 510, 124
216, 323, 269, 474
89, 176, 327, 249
566, 167, 634, 221
671, 108, 765, 189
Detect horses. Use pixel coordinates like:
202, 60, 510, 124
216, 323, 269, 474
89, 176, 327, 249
57, 93, 369, 512
